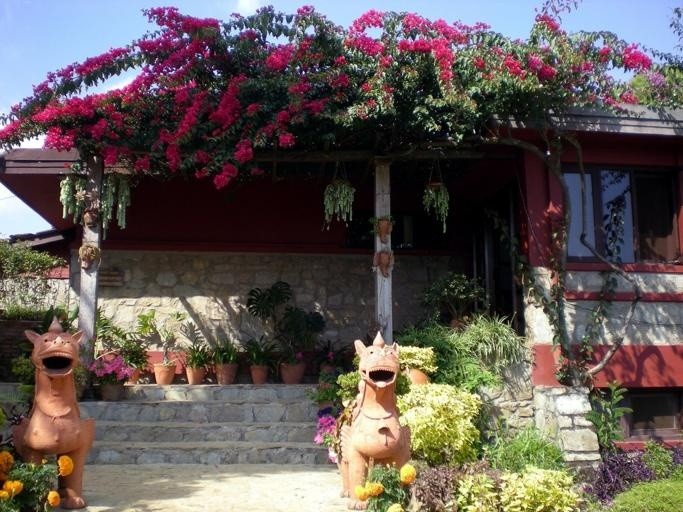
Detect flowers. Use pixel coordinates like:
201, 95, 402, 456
305, 339, 341, 464
79, 335, 134, 382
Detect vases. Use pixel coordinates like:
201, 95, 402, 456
101, 383, 121, 401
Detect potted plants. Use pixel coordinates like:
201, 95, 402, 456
74, 189, 103, 269
367, 214, 396, 277
134, 282, 326, 383
423, 181, 450, 233
322, 178, 356, 231
421, 269, 489, 329
